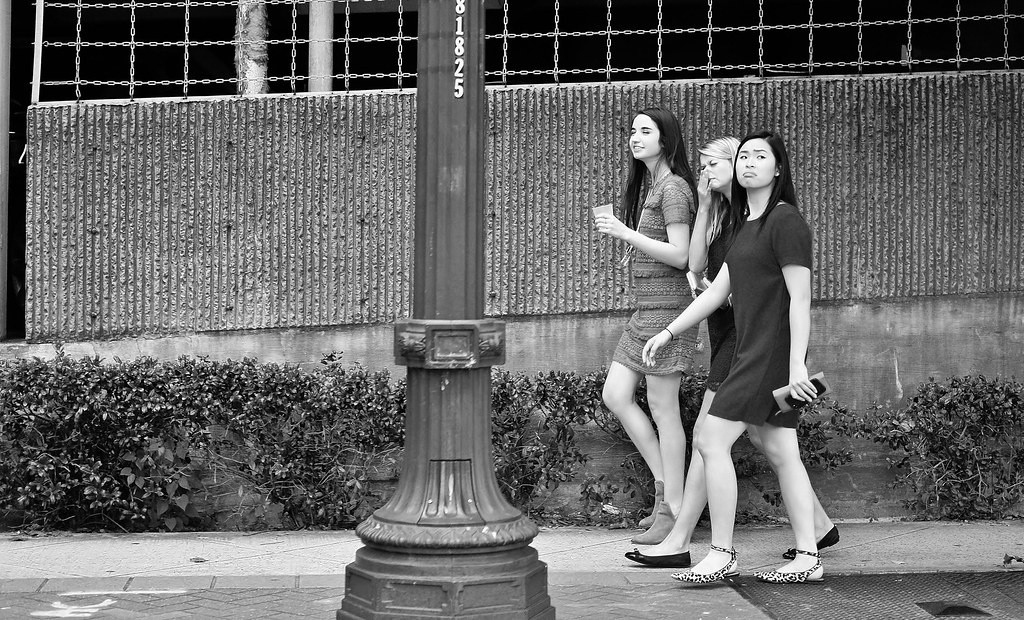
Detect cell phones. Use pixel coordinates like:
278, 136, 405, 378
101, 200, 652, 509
785, 378, 826, 410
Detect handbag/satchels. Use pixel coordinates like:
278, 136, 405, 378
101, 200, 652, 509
685, 269, 733, 310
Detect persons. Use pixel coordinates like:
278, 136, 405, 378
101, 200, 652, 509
596, 108, 700, 544
672, 131, 828, 587
626, 134, 841, 569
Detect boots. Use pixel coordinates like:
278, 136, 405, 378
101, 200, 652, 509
638, 479, 665, 527
632, 500, 678, 545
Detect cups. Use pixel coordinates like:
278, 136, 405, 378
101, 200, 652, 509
592, 203, 613, 219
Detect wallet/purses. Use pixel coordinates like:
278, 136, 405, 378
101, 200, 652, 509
772, 371, 832, 416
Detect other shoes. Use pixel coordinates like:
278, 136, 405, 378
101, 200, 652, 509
782, 524, 839, 559
754, 550, 825, 584
672, 545, 738, 583
625, 548, 690, 567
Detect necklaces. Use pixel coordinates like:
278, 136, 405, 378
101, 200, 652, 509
619, 168, 673, 269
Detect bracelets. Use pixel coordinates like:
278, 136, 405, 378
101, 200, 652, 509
665, 327, 675, 341
